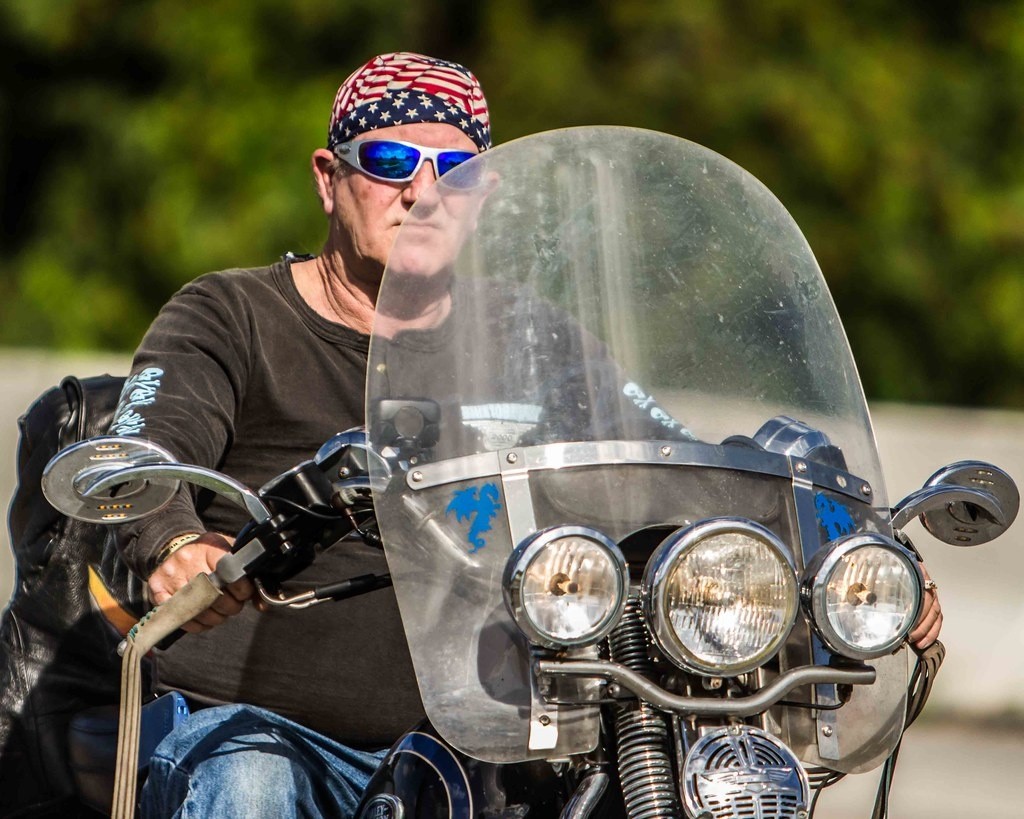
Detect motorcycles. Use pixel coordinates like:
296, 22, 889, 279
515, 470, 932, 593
0, 124, 1020, 819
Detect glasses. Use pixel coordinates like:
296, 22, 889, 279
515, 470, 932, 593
333, 139, 487, 191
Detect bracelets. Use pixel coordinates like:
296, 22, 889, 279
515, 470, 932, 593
155, 533, 201, 565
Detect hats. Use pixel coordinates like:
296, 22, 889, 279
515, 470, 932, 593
328, 51, 492, 153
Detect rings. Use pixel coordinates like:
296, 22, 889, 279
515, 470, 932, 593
922, 580, 938, 590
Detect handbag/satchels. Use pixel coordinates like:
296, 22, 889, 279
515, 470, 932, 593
0, 374, 129, 742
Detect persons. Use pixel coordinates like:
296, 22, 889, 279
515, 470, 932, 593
101, 47, 946, 818
